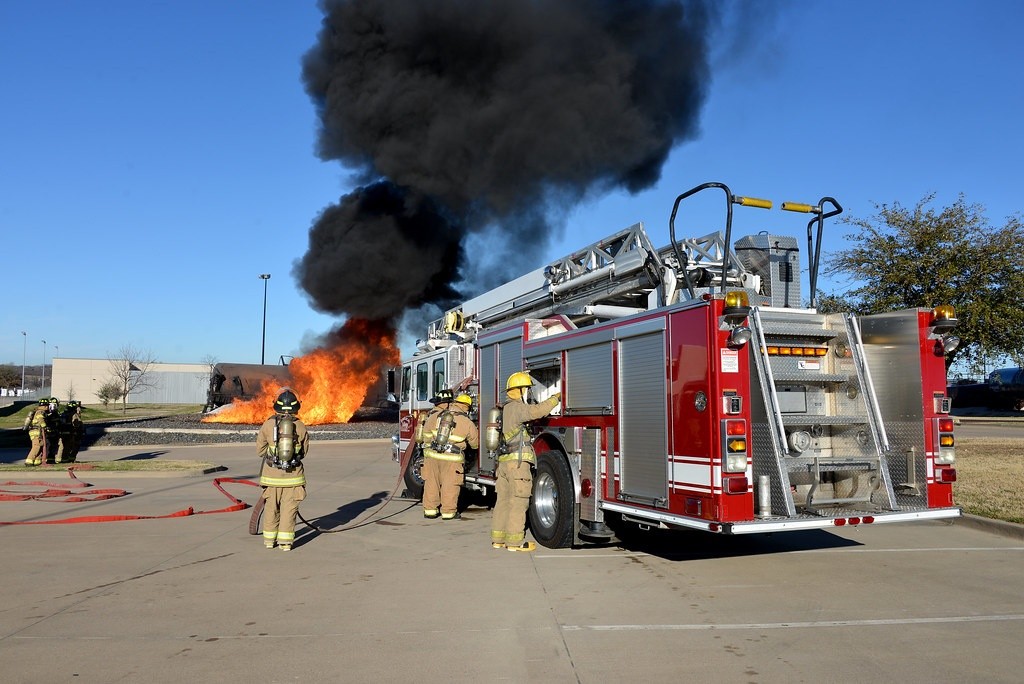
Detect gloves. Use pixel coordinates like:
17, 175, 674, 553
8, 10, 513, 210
551, 392, 561, 401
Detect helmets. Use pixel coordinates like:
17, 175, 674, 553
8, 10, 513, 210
39, 398, 50, 406
65, 400, 77, 410
429, 389, 453, 403
453, 394, 473, 406
49, 398, 59, 403
501, 372, 536, 393
273, 391, 300, 413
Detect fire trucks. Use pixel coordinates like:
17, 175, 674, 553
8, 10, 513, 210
391, 181, 963, 549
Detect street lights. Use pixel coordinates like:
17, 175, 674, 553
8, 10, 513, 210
21, 331, 26, 400
41, 340, 46, 390
258, 274, 270, 365
54, 346, 60, 358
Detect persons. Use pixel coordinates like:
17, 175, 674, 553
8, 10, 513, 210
421, 388, 479, 521
23, 397, 84, 467
490, 373, 561, 553
255, 390, 310, 550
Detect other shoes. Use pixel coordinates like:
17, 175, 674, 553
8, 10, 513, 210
442, 512, 462, 521
424, 513, 441, 520
507, 542, 535, 552
493, 542, 505, 549
266, 545, 274, 548
46, 459, 57, 464
279, 544, 292, 551
25, 463, 34, 467
